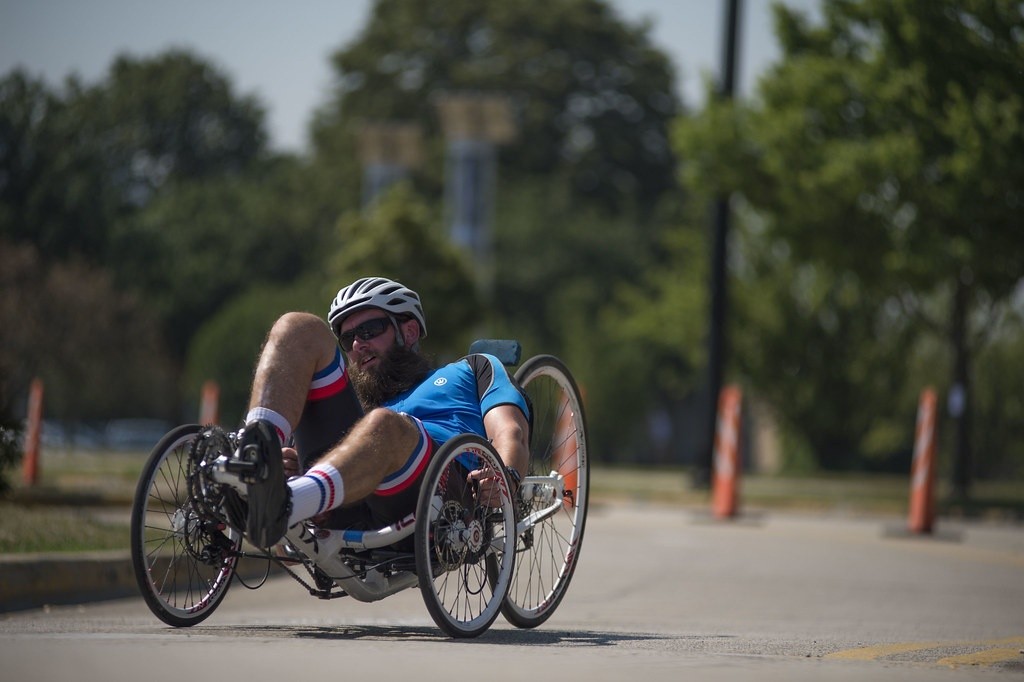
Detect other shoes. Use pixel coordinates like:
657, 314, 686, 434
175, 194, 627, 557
226, 486, 249, 533
239, 419, 294, 549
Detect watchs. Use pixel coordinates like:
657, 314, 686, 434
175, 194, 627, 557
503, 464, 521, 496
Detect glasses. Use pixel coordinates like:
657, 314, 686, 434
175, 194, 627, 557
340, 316, 408, 353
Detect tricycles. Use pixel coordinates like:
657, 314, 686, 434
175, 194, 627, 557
129, 336, 592, 638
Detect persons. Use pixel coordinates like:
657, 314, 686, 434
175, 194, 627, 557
209, 277, 534, 550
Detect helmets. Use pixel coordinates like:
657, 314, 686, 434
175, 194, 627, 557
328, 276, 427, 339
